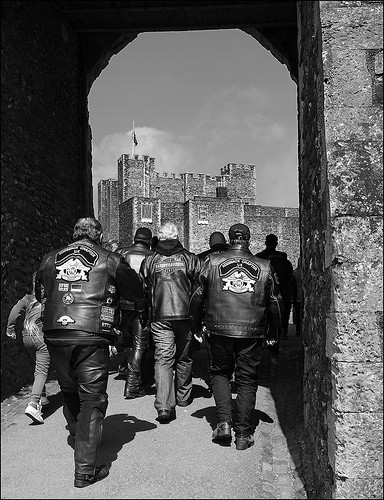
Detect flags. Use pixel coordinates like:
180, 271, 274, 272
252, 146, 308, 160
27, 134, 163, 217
132, 120, 138, 146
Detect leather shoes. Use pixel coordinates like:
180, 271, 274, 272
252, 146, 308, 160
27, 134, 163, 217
73, 464, 109, 488
156, 409, 175, 424
211, 421, 232, 441
123, 388, 145, 399
234, 434, 254, 449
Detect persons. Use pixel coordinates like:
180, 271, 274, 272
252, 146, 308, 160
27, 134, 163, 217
6, 272, 51, 424
100, 223, 303, 424
34, 217, 143, 487
187, 223, 283, 450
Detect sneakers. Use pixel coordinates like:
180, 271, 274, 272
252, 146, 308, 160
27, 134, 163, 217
37, 394, 48, 405
24, 398, 45, 425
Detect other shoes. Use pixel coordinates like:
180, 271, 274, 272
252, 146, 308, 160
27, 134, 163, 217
228, 222, 250, 241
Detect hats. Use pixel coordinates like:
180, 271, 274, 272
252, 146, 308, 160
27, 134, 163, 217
134, 228, 152, 240
